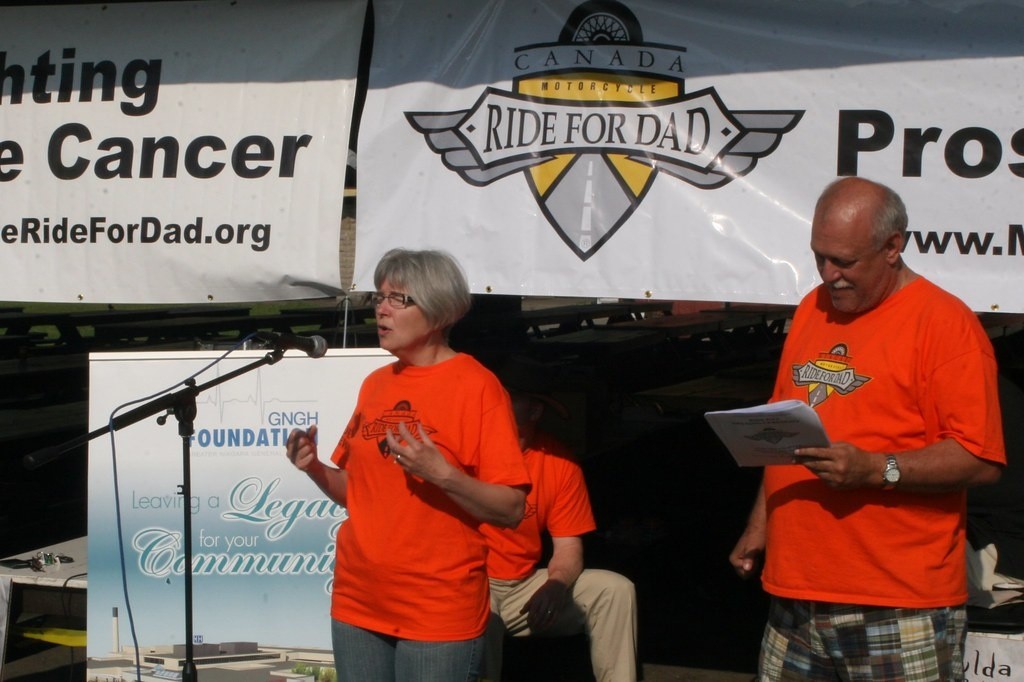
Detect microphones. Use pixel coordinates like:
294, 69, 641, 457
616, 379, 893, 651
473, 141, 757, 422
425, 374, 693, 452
257, 329, 328, 358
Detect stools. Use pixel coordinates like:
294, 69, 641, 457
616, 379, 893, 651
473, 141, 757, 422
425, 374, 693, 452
499, 633, 595, 682
966, 603, 1024, 633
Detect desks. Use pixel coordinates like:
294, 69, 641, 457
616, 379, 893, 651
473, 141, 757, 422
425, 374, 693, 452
0, 535, 90, 682
0, 306, 786, 489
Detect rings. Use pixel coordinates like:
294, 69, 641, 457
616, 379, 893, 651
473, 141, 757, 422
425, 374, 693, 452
396, 455, 402, 461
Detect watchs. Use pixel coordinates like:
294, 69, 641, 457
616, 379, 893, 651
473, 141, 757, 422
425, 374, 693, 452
881, 453, 900, 490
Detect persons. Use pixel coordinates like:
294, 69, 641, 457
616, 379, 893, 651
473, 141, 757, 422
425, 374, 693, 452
286, 250, 533, 682
477, 357, 639, 681
964, 373, 1024, 609
724, 178, 1007, 681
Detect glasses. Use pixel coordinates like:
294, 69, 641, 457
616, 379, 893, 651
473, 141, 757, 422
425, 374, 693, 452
370, 291, 420, 307
